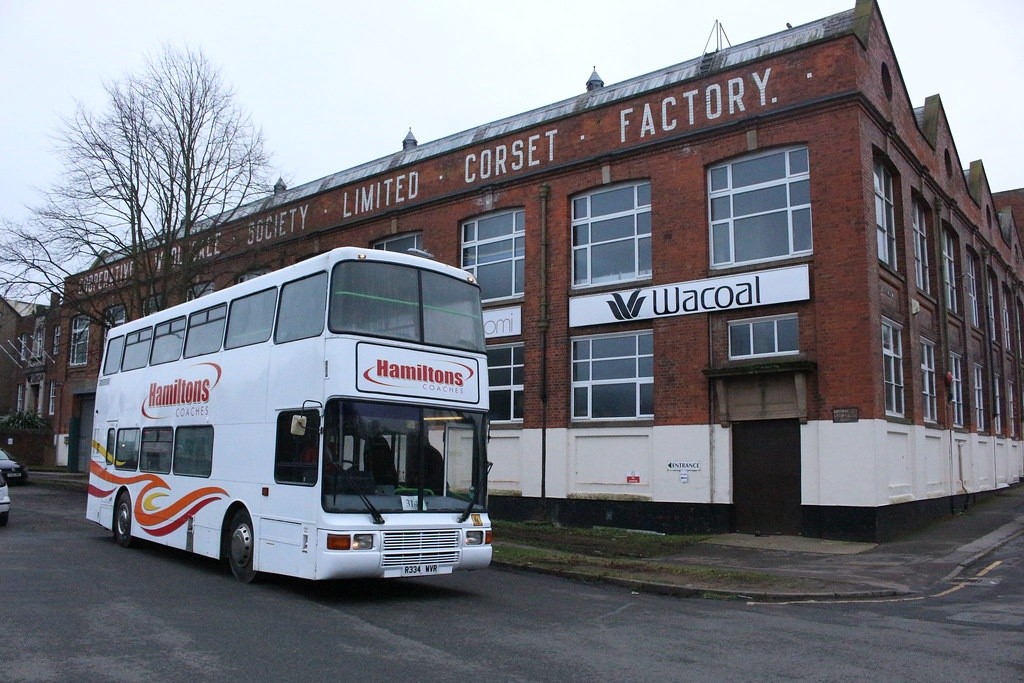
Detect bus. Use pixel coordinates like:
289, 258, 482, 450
84, 245, 496, 587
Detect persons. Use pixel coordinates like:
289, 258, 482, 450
363, 420, 397, 486
304, 437, 330, 464
414, 435, 444, 496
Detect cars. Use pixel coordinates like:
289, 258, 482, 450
0, 450, 28, 522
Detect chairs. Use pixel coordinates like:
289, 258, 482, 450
364, 436, 399, 486
